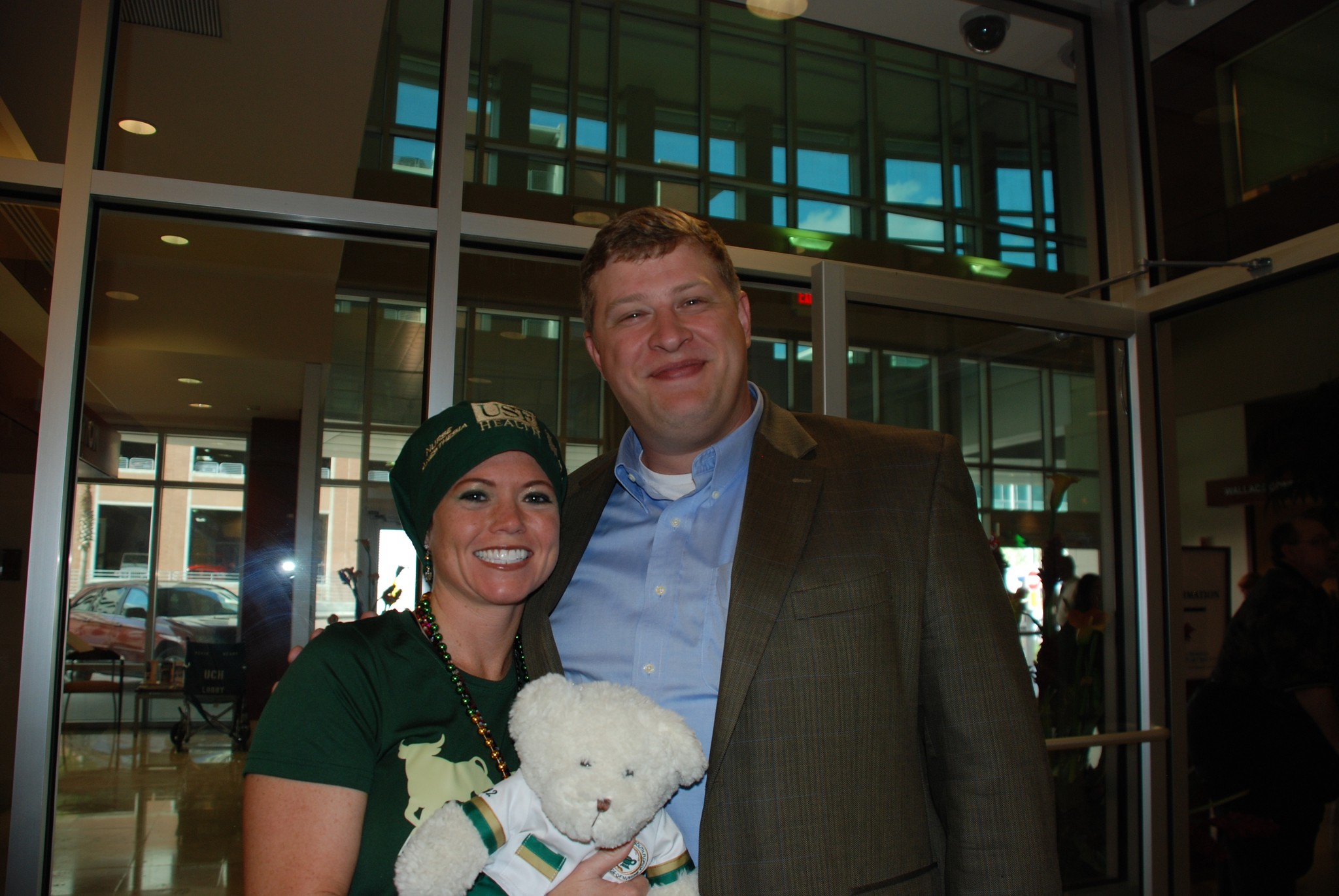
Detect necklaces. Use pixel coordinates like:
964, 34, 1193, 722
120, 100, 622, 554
413, 589, 531, 781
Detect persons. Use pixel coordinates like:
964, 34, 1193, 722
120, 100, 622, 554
1036, 570, 1111, 818
1200, 509, 1339, 895
489, 203, 1061, 894
238, 397, 572, 895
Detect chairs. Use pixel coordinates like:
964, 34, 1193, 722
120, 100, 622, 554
170, 638, 251, 751
61, 648, 125, 734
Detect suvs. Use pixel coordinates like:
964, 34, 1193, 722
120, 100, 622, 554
63, 578, 239, 681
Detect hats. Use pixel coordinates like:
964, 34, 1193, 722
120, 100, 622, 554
389, 399, 568, 584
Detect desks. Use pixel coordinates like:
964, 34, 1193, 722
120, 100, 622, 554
134, 683, 186, 736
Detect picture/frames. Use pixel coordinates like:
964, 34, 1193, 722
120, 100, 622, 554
1178, 545, 1233, 681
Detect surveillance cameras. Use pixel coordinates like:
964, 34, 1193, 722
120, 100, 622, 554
957, 5, 1011, 53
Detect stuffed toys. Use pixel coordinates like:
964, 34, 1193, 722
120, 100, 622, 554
393, 671, 711, 896
